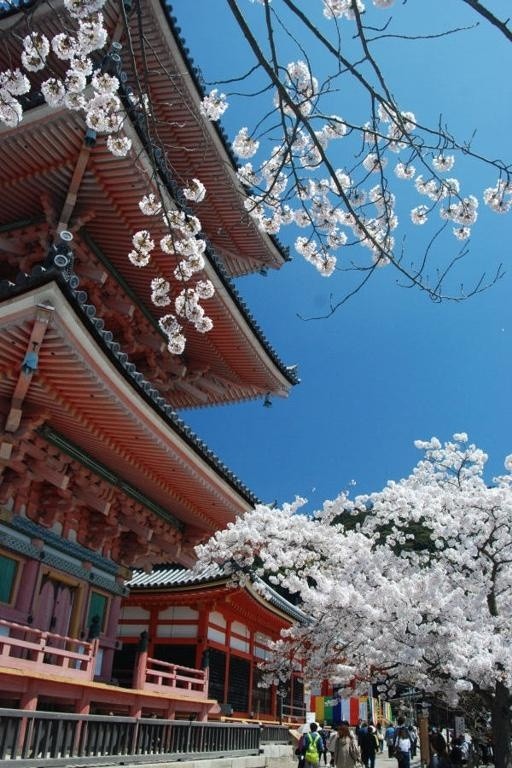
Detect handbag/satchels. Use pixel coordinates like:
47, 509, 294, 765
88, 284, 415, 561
350, 740, 360, 760
393, 745, 404, 762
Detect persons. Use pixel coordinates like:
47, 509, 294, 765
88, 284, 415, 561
295, 714, 474, 768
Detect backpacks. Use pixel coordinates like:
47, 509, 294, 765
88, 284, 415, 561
305, 733, 320, 763
